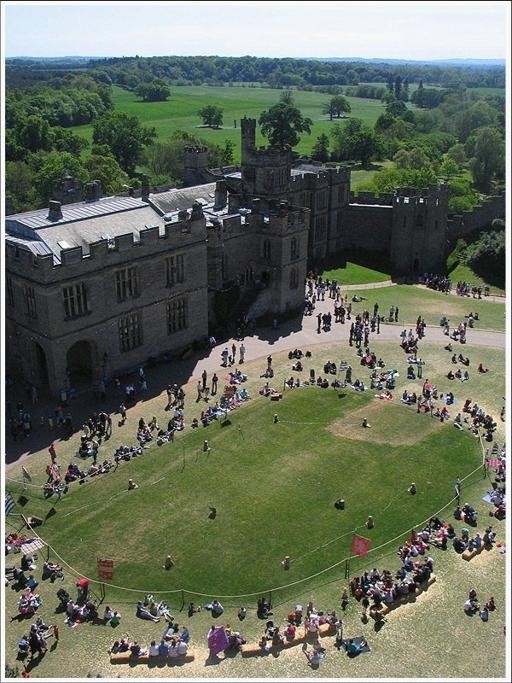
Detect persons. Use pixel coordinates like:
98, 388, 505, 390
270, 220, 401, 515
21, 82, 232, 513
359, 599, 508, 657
396, 403, 505, 598
285, 265, 505, 403
486, 596, 495, 609
463, 598, 478, 612
468, 588, 477, 601
479, 607, 488, 621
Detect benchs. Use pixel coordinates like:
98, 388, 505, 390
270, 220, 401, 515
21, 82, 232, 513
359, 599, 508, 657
110, 646, 194, 663
240, 622, 333, 655
370, 572, 435, 619
462, 536, 484, 560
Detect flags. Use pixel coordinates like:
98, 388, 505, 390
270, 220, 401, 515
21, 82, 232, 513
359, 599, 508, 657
353, 532, 371, 557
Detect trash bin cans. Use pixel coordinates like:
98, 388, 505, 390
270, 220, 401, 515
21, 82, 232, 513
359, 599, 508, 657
273, 319, 278, 328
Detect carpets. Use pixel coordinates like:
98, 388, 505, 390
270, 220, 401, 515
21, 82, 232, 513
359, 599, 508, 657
20, 536, 45, 555
